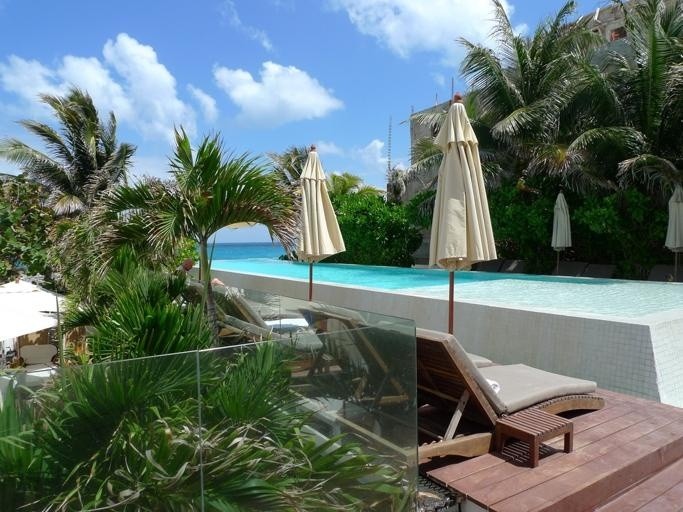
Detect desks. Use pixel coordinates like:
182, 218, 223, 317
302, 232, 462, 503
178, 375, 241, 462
496, 407, 573, 467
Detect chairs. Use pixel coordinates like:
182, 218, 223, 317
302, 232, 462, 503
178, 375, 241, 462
7, 327, 109, 408
211, 282, 340, 378
354, 320, 602, 473
300, 306, 494, 420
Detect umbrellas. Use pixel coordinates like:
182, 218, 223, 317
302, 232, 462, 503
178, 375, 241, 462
428, 91, 498, 334
665, 182, 683, 282
296, 144, 346, 301
551, 190, 572, 274
